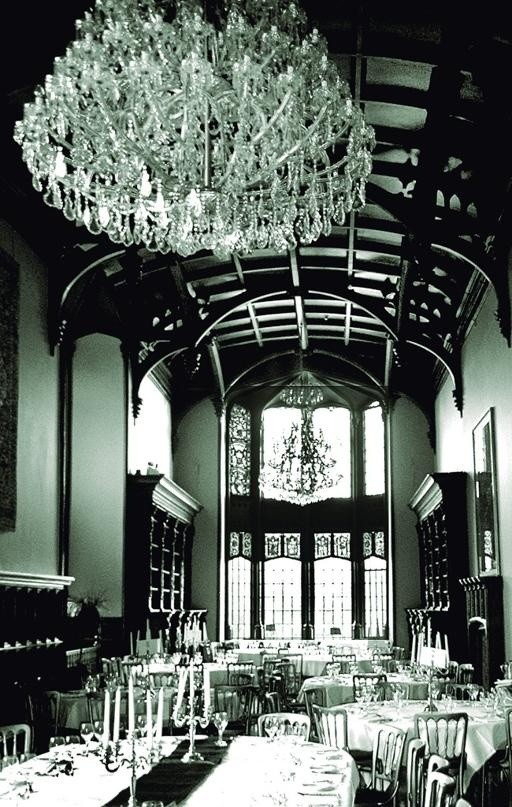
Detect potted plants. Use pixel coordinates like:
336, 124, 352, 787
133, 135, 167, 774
67, 586, 111, 640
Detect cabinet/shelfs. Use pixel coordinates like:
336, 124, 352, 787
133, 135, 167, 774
123, 473, 208, 650
407, 472, 468, 670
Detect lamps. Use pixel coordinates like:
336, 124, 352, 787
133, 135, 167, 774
13, 0, 376, 261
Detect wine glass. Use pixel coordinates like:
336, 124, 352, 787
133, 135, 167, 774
2, 651, 480, 779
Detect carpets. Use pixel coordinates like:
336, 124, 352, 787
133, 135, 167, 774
268, 322, 344, 496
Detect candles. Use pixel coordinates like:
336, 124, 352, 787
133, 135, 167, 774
130, 618, 164, 657
410, 618, 450, 667
183, 614, 207, 644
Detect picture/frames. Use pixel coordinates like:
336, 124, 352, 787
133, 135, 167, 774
471, 407, 502, 576
